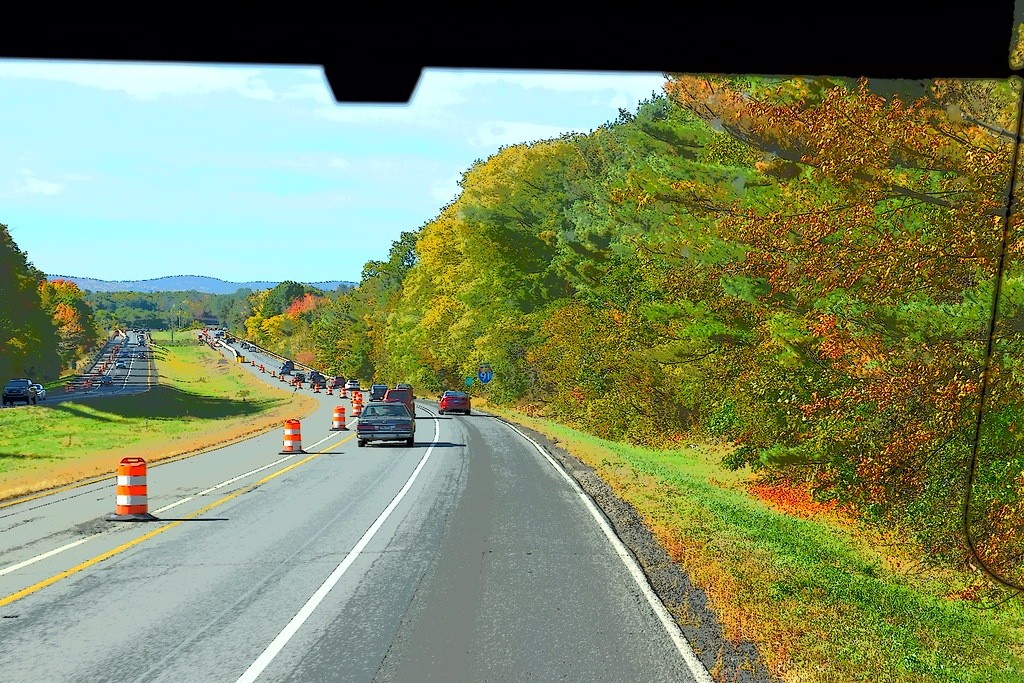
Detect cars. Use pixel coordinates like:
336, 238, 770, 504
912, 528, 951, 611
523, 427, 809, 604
437, 390, 472, 415
369, 384, 388, 401
282, 360, 294, 370
395, 383, 413, 399
280, 365, 291, 375
356, 401, 417, 448
307, 370, 320, 380
33, 383, 46, 401
381, 388, 416, 418
132, 327, 147, 348
136, 353, 146, 359
294, 371, 306, 382
122, 350, 130, 358
213, 329, 257, 353
344, 379, 361, 391
100, 376, 113, 385
115, 359, 126, 369
310, 374, 326, 390
330, 376, 345, 389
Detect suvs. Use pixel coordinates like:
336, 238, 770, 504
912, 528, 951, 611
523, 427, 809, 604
2, 378, 37, 405
